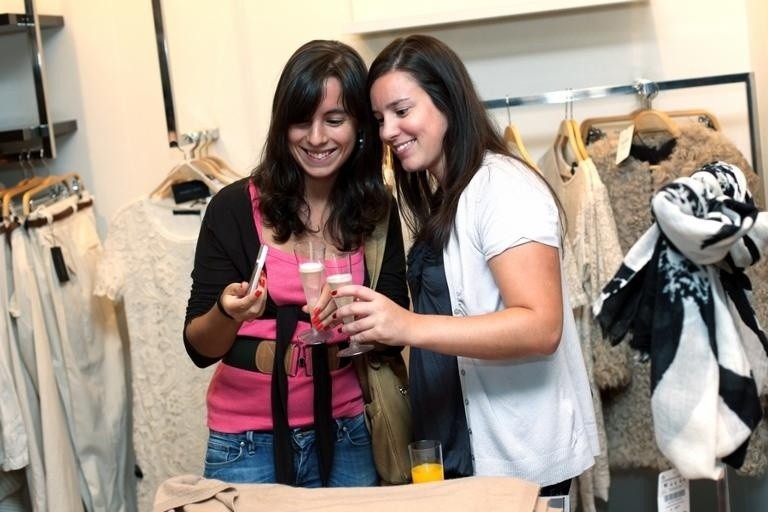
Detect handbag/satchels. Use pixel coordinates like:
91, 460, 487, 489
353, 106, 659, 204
352, 345, 418, 486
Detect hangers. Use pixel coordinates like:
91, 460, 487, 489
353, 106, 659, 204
382, 77, 677, 178
0, 144, 86, 228
149, 126, 249, 200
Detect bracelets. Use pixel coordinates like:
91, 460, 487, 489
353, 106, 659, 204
212, 291, 235, 319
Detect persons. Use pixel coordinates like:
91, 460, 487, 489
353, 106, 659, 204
179, 40, 411, 487
327, 30, 604, 510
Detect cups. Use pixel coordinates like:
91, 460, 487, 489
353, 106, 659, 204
407, 440, 445, 484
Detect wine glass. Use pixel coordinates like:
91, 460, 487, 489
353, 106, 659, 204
319, 252, 374, 357
293, 239, 334, 345
